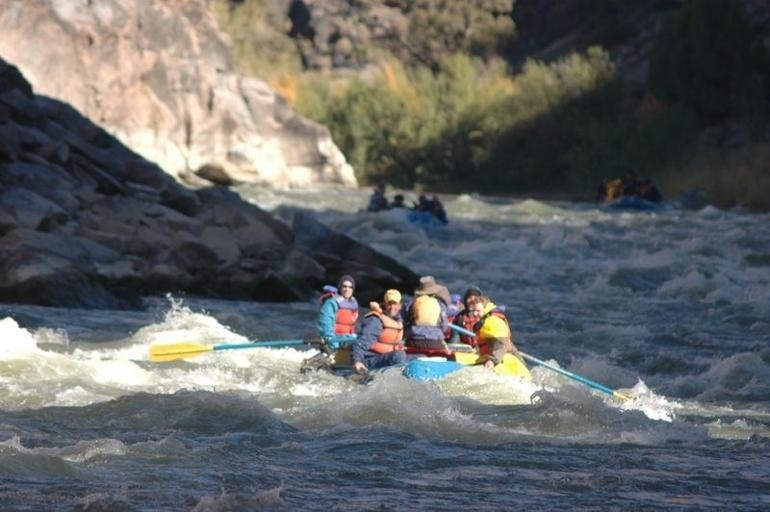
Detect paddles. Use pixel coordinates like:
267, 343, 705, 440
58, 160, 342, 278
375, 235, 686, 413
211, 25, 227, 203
150, 337, 355, 363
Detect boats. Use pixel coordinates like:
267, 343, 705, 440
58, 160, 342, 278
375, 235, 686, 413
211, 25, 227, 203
599, 195, 662, 215
298, 343, 532, 385
401, 208, 447, 229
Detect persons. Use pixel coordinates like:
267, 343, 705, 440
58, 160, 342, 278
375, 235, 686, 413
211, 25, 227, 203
595, 166, 663, 207
314, 274, 513, 375
368, 183, 446, 221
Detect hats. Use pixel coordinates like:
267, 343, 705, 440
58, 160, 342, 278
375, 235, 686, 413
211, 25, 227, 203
465, 285, 483, 296
383, 289, 401, 303
414, 276, 451, 307
337, 275, 355, 289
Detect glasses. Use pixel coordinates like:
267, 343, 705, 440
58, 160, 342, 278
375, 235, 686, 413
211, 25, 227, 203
342, 285, 353, 288
